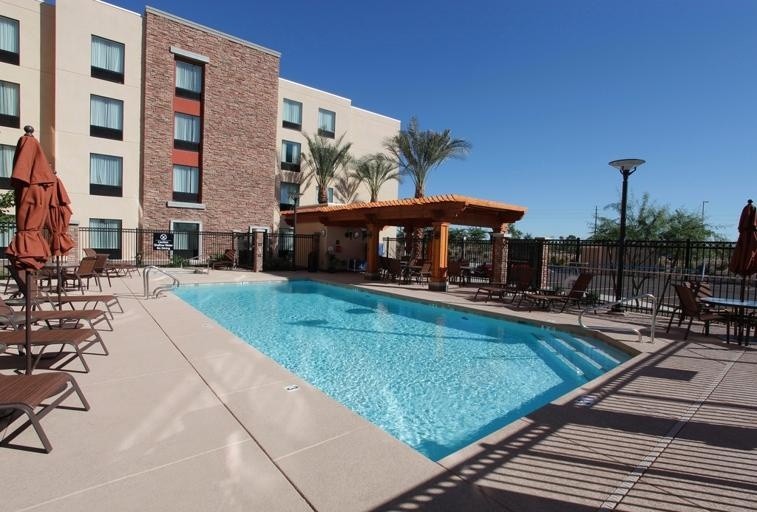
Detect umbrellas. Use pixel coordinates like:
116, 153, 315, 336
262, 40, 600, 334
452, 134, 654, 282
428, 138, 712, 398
2, 124, 57, 376
728, 199, 756, 346
44, 175, 75, 325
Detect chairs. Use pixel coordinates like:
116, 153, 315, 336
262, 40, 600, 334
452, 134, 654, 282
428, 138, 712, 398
665, 275, 757, 347
0, 248, 141, 453
213, 248, 237, 270
377, 255, 595, 313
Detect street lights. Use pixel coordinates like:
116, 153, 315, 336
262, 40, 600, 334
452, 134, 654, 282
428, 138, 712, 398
460, 229, 468, 262
702, 201, 711, 220
608, 156, 646, 316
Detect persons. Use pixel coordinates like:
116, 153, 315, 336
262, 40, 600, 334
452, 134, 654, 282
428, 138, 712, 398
408, 252, 420, 273
